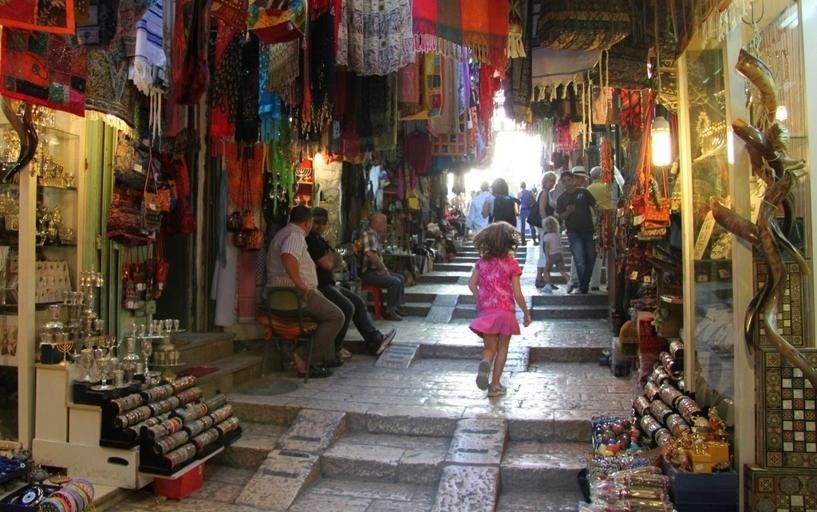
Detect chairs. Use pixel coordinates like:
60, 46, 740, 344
262, 286, 318, 384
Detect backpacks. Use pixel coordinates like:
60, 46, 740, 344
489, 193, 517, 226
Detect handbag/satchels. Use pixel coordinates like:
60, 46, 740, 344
630, 187, 672, 241
120, 237, 168, 310
226, 210, 264, 250
107, 127, 177, 245
527, 190, 555, 228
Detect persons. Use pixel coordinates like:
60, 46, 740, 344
464, 166, 621, 294
262, 205, 346, 377
468, 220, 532, 397
305, 206, 397, 360
360, 212, 408, 321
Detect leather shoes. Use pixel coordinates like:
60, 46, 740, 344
322, 357, 343, 368
395, 308, 409, 316
298, 365, 332, 378
382, 311, 403, 321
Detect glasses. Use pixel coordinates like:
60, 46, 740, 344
314, 220, 326, 225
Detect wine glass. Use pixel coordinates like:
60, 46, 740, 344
81, 348, 138, 391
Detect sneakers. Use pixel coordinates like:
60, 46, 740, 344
375, 328, 396, 356
476, 360, 490, 390
488, 381, 507, 397
335, 348, 353, 359
536, 280, 589, 293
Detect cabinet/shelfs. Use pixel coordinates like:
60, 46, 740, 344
1, 107, 242, 498
341, 108, 463, 320
578, 0, 817, 512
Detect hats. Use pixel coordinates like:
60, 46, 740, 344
572, 166, 591, 179
311, 206, 328, 221
